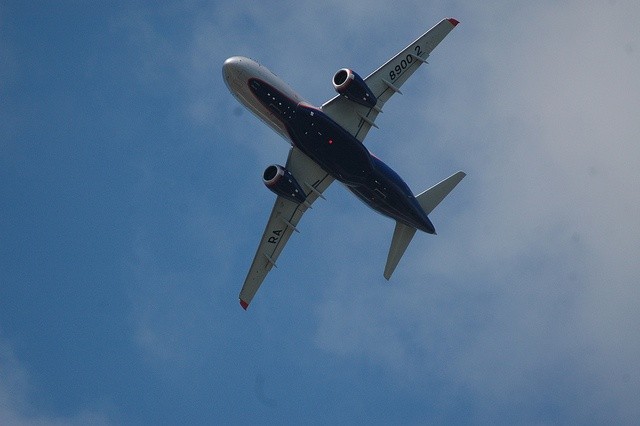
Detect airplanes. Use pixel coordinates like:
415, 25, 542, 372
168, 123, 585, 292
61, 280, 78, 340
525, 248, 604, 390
222, 17, 467, 311
